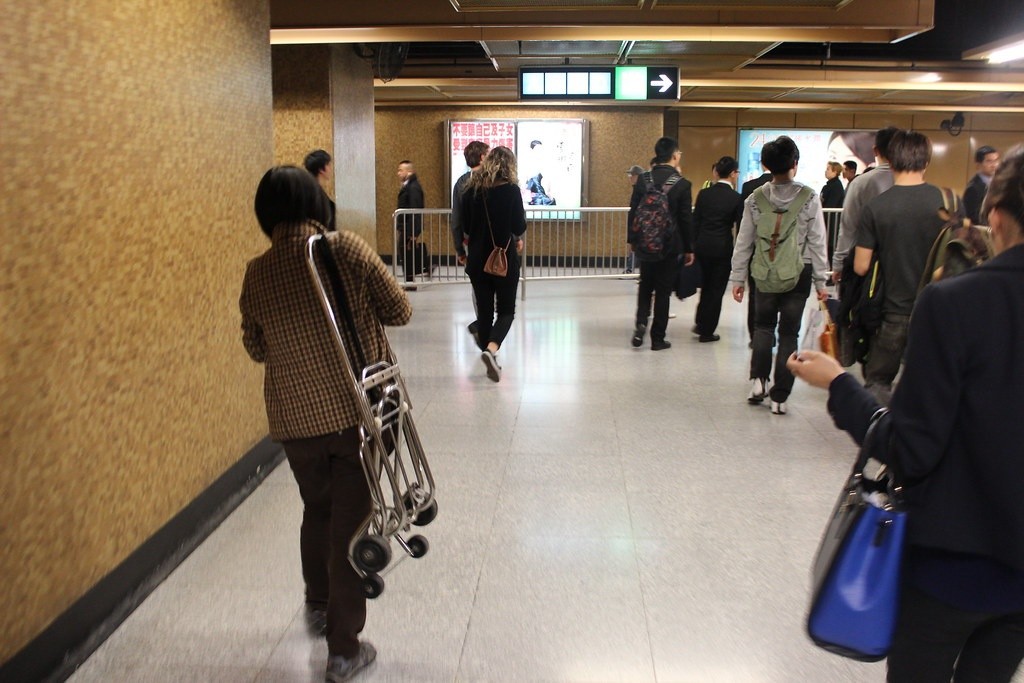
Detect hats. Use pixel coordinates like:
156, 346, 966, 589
626, 166, 643, 175
824, 299, 858, 329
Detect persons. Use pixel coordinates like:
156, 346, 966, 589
239, 166, 413, 683
518, 140, 560, 206
787, 147, 1024, 683
627, 126, 998, 413
303, 151, 336, 232
394, 160, 425, 290
449, 141, 527, 382
825, 131, 877, 191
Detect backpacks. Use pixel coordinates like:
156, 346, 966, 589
631, 172, 683, 253
908, 180, 988, 334
749, 183, 815, 293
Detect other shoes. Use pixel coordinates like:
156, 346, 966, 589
693, 325, 720, 342
468, 321, 482, 348
632, 323, 645, 347
325, 641, 377, 683
747, 377, 787, 414
480, 347, 502, 383
308, 611, 327, 636
651, 340, 671, 350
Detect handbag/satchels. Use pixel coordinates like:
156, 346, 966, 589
795, 299, 856, 368
404, 239, 429, 273
807, 407, 902, 662
484, 246, 508, 277
